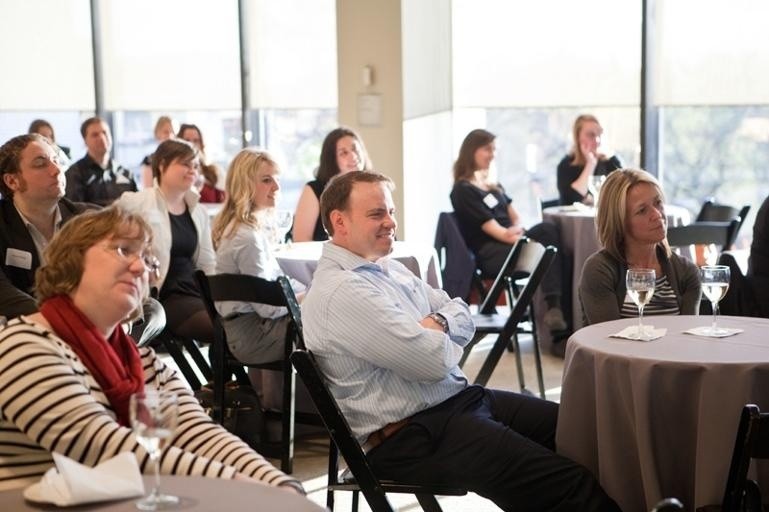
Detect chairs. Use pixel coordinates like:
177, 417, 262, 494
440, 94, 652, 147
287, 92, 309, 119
542, 199, 564, 208
667, 200, 753, 315
194, 268, 346, 473
436, 207, 530, 357
453, 236, 560, 401
149, 335, 215, 388
648, 401, 769, 512
290, 345, 471, 510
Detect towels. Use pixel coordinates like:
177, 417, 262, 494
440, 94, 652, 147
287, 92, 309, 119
23, 451, 147, 507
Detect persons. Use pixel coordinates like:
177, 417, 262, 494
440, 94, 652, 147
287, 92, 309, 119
0, 206, 326, 510
557, 114, 620, 202
0, 115, 373, 347
450, 128, 568, 332
577, 167, 703, 327
300, 170, 622, 511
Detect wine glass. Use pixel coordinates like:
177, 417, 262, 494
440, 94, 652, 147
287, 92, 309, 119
697, 266, 731, 336
625, 267, 657, 339
588, 174, 606, 213
129, 388, 183, 511
276, 210, 294, 249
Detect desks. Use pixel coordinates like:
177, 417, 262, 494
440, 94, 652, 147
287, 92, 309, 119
525, 205, 698, 357
195, 201, 297, 246
0, 474, 331, 512
551, 314, 769, 512
266, 238, 445, 293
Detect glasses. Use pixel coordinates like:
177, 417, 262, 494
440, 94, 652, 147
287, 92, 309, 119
86, 241, 161, 278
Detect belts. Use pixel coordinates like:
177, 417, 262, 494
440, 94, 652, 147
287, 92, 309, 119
360, 418, 411, 455
